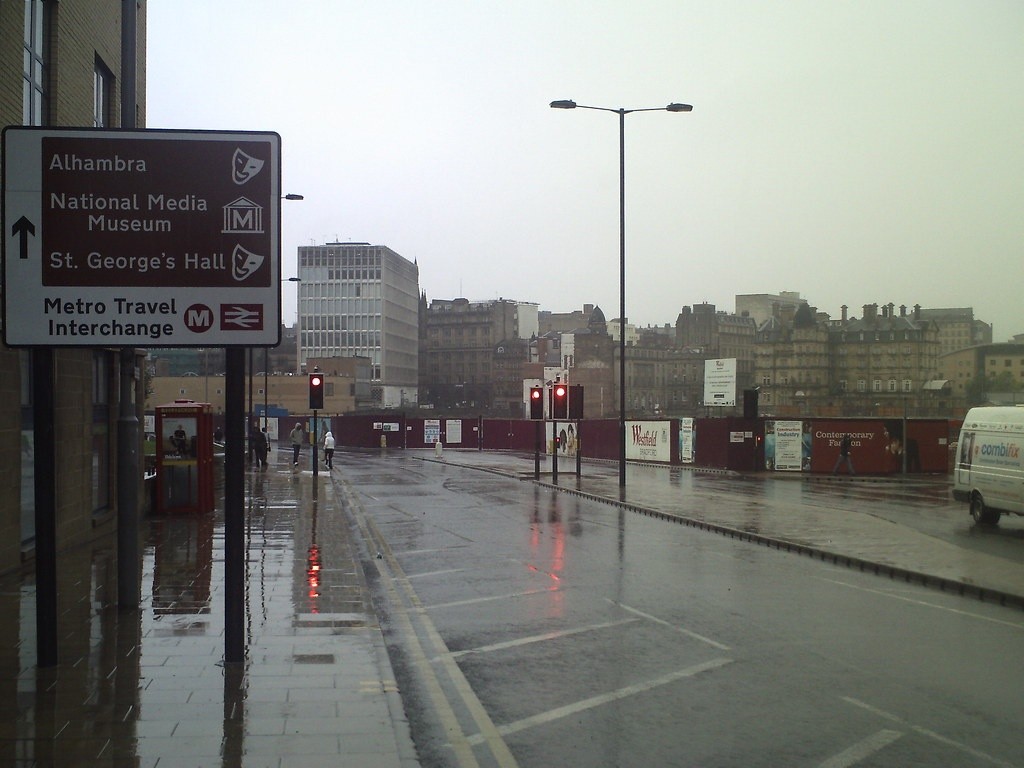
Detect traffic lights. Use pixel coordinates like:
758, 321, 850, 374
307, 546, 322, 596
530, 386, 544, 421
552, 383, 569, 420
309, 373, 325, 409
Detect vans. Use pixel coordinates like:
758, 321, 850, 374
953, 404, 1024, 525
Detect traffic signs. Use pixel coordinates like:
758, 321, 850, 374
0, 125, 282, 348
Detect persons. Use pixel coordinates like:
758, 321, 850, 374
213, 426, 223, 442
251, 427, 270, 472
289, 422, 304, 467
883, 420, 922, 473
560, 424, 574, 456
323, 431, 335, 469
304, 418, 310, 443
144, 433, 153, 441
833, 436, 855, 476
173, 425, 189, 460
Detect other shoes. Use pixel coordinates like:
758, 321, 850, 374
295, 462, 298, 465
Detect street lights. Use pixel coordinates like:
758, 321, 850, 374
265, 276, 301, 433
549, 100, 694, 481
248, 194, 304, 462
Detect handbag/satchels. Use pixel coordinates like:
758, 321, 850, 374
269, 447, 271, 452
